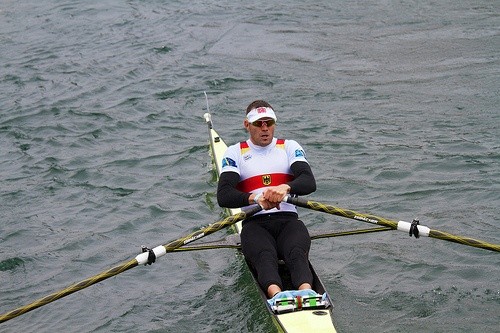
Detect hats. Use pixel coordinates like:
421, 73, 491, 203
245, 106, 278, 124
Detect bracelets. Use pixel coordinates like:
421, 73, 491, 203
253, 194, 262, 204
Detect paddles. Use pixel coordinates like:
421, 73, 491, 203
282, 196, 500, 252
0, 204, 264, 324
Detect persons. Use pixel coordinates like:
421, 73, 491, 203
217, 101, 317, 297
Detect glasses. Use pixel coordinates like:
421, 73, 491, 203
252, 120, 275, 127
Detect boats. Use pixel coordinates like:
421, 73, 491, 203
201, 111, 340, 333
